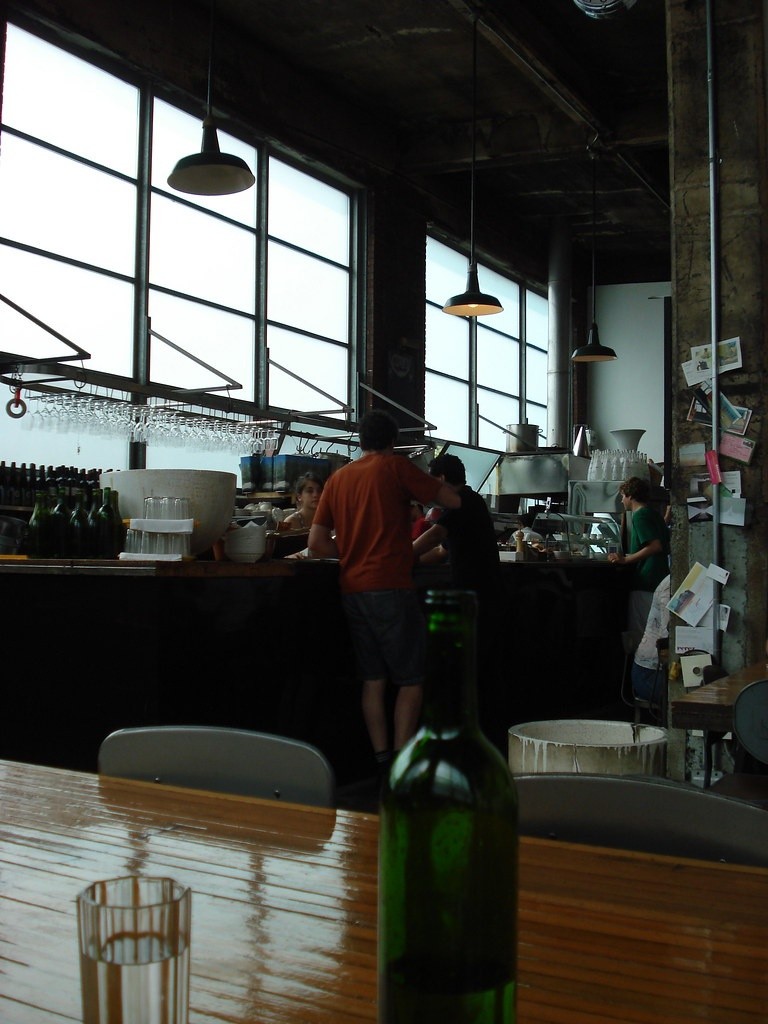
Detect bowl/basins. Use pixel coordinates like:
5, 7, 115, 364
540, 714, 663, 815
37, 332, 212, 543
244, 503, 256, 510
553, 551, 570, 557
553, 534, 575, 540
225, 526, 265, 564
594, 553, 609, 561
100, 468, 237, 554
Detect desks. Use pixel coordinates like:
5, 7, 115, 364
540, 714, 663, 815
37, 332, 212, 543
0, 757, 768, 1024
669, 661, 768, 789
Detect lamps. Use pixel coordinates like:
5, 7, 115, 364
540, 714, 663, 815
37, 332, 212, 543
440, 5, 503, 313
173, 0, 254, 193
569, 144, 617, 362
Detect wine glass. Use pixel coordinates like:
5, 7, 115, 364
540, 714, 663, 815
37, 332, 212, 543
24, 391, 279, 451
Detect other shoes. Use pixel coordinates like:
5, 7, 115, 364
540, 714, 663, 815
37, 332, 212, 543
374, 750, 397, 776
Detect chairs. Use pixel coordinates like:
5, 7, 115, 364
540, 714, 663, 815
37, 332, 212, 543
96, 677, 768, 867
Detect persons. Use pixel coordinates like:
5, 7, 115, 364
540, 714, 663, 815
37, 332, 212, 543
631, 574, 670, 707
283, 472, 443, 540
608, 476, 670, 645
413, 452, 504, 732
507, 514, 544, 543
308, 410, 461, 774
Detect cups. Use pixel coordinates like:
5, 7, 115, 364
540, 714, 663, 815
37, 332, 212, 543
77, 875, 193, 1024
144, 497, 190, 520
126, 529, 193, 555
598, 534, 603, 538
583, 534, 589, 538
591, 534, 597, 538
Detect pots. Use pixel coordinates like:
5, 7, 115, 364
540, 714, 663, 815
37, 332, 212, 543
505, 424, 543, 454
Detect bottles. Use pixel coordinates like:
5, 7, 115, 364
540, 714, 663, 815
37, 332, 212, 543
380, 591, 515, 1024
0, 460, 124, 563
588, 449, 651, 483
